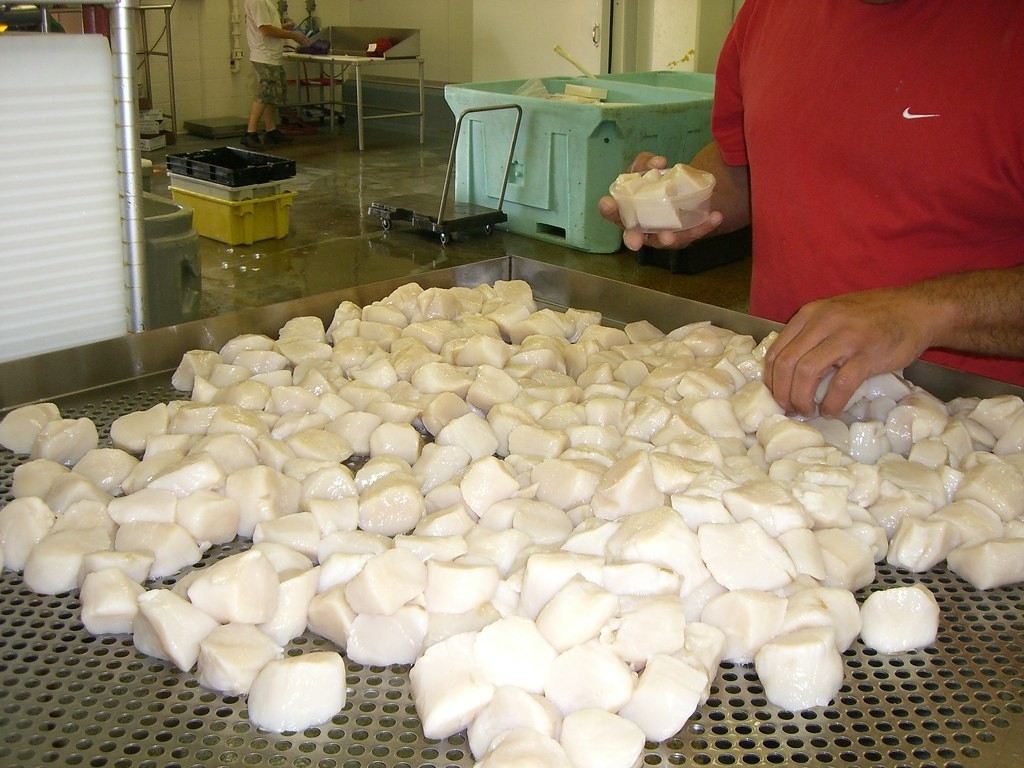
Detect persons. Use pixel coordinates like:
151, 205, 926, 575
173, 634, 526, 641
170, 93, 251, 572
239, 0, 311, 152
598, 0, 1024, 420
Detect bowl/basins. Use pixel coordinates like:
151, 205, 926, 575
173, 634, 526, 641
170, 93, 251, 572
608, 169, 716, 233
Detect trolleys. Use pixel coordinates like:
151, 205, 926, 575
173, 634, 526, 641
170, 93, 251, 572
367, 102, 522, 243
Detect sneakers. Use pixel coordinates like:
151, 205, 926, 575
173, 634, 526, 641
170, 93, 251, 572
263, 129, 294, 144
240, 132, 265, 151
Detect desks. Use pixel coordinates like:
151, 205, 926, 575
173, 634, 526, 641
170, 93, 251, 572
282, 51, 425, 150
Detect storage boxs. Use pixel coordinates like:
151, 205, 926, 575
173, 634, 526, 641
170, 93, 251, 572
444, 76, 713, 255
581, 70, 717, 97
637, 225, 754, 274
166, 145, 297, 246
